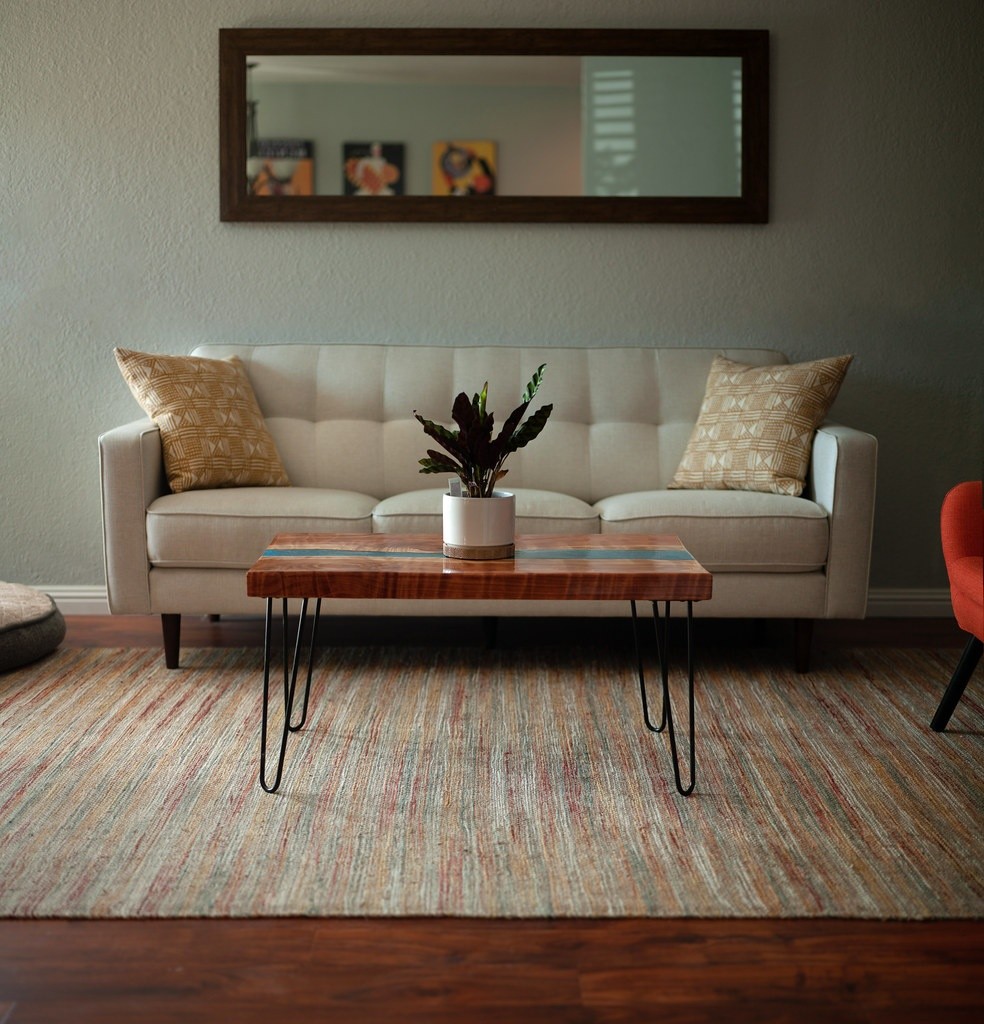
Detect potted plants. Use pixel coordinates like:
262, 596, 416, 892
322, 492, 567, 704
413, 361, 553, 559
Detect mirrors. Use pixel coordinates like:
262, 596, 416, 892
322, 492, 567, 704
219, 27, 768, 225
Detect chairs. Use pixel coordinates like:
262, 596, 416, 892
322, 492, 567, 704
930, 480, 984, 733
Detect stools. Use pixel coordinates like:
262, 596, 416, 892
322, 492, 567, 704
0, 581, 65, 675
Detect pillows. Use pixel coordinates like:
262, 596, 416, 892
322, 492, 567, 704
667, 350, 852, 498
114, 348, 291, 493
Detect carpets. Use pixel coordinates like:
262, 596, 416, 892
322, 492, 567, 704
1, 643, 982, 919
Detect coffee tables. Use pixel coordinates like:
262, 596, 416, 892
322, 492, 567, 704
247, 531, 713, 796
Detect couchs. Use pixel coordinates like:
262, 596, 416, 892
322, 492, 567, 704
98, 345, 877, 670
